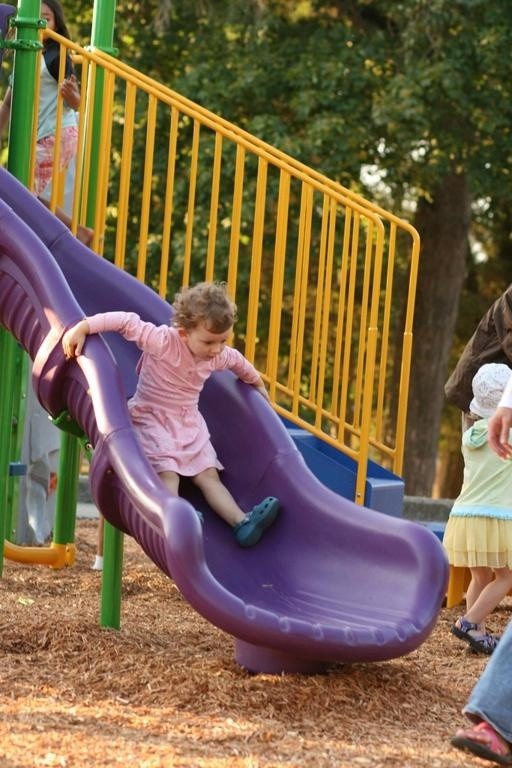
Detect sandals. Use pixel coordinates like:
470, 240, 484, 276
450, 616, 500, 655
233, 496, 280, 548
451, 720, 512, 766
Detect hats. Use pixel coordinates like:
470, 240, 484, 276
469, 363, 512, 419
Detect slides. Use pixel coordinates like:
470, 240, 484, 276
2, 169, 449, 674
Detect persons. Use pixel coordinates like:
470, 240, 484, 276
446, 367, 510, 768
438, 361, 511, 655
2, 0, 96, 246
440, 279, 512, 608
59, 281, 284, 549
